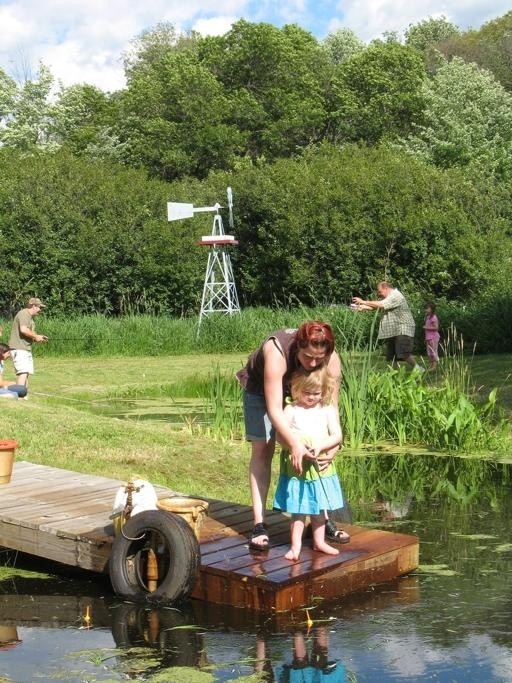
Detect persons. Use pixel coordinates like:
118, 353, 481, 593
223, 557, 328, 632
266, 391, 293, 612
0, 341, 27, 399
255, 555, 345, 683
8, 297, 48, 399
234, 321, 350, 551
422, 301, 440, 369
275, 363, 343, 561
0, 323, 4, 336
350, 282, 425, 374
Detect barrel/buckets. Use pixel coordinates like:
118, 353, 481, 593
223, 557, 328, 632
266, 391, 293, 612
0, 440, 19, 485
156, 496, 209, 546
113, 478, 159, 520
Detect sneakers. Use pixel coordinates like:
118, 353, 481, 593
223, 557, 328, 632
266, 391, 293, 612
418, 366, 437, 373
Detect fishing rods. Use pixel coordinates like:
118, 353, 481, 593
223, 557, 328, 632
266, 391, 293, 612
28, 391, 109, 404
48, 338, 121, 339
313, 461, 342, 543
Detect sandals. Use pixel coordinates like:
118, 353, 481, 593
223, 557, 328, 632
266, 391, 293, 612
251, 523, 269, 550
306, 518, 350, 543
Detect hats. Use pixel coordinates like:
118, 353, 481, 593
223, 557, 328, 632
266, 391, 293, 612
28, 298, 46, 308
